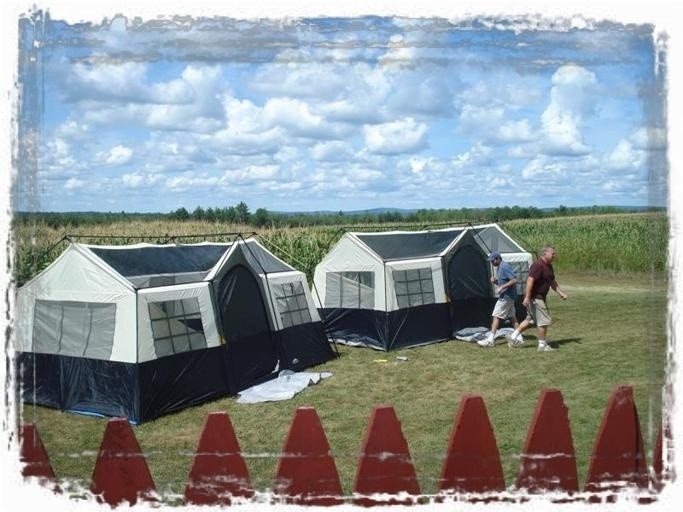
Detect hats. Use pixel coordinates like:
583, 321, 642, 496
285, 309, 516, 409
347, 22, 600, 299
485, 251, 500, 261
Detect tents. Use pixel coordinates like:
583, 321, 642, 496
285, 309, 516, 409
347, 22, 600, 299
312, 218, 534, 353
15, 230, 336, 425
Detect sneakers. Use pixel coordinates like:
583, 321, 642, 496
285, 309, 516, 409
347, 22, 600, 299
516, 334, 524, 344
477, 337, 494, 347
536, 343, 555, 351
507, 335, 516, 348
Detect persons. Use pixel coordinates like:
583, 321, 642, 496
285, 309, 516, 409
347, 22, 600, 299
506, 245, 568, 352
477, 252, 523, 346
